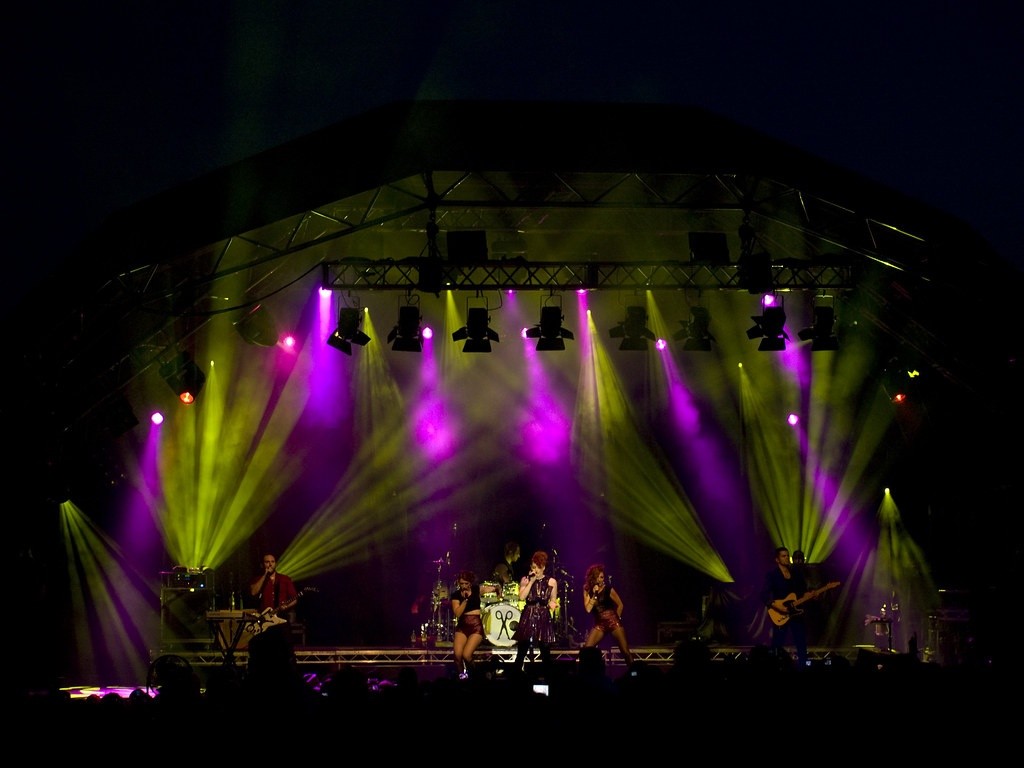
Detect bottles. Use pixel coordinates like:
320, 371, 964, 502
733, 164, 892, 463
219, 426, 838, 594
228, 595, 235, 610
239, 595, 244, 610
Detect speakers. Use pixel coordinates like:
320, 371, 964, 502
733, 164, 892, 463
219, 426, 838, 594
161, 587, 216, 642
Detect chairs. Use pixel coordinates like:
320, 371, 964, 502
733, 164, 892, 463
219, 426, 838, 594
288, 608, 307, 648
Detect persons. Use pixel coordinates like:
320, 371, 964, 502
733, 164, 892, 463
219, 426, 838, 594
250, 550, 298, 662
759, 546, 819, 668
511, 551, 558, 666
0, 631, 1024, 768
450, 569, 486, 664
583, 564, 634, 667
493, 541, 520, 589
792, 550, 822, 591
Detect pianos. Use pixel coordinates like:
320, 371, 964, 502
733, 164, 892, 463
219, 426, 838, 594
205, 609, 257, 668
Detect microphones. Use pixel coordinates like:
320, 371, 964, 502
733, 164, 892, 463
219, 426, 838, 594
528, 573, 535, 578
463, 588, 469, 601
561, 570, 573, 579
594, 584, 599, 599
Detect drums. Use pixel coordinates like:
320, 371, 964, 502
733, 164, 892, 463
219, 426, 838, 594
503, 580, 524, 602
548, 597, 561, 623
508, 599, 527, 611
433, 586, 450, 606
481, 602, 522, 648
479, 581, 502, 603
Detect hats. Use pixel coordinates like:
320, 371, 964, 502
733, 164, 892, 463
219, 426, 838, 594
793, 550, 804, 557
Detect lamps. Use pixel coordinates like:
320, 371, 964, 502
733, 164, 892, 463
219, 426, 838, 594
452, 290, 502, 354
234, 303, 278, 348
608, 289, 657, 352
672, 289, 718, 352
525, 288, 575, 353
327, 291, 371, 356
747, 292, 790, 352
876, 367, 909, 407
732, 224, 775, 294
147, 328, 206, 406
799, 288, 841, 352
387, 289, 424, 353
684, 231, 731, 266
444, 229, 489, 265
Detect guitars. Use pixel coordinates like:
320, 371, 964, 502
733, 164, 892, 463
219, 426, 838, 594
245, 585, 320, 636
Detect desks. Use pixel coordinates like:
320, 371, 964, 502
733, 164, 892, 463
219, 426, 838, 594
655, 621, 698, 644
